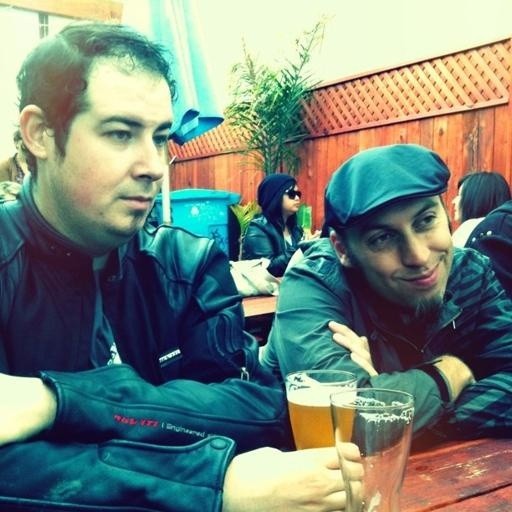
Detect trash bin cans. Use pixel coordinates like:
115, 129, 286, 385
155, 190, 240, 260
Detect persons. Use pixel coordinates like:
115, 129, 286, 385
449, 170, 512, 251
259, 142, 512, 455
241, 174, 325, 279
0, 131, 31, 206
1, 22, 364, 512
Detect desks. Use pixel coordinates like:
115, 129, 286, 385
358, 426, 512, 510
242, 275, 281, 342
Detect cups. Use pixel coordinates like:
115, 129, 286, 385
285, 369, 358, 451
329, 387, 415, 512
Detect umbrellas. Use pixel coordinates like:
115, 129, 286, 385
118, 0, 225, 229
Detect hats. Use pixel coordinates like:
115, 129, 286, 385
257, 173, 296, 216
324, 144, 451, 231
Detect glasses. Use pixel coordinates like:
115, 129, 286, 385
284, 189, 302, 199
